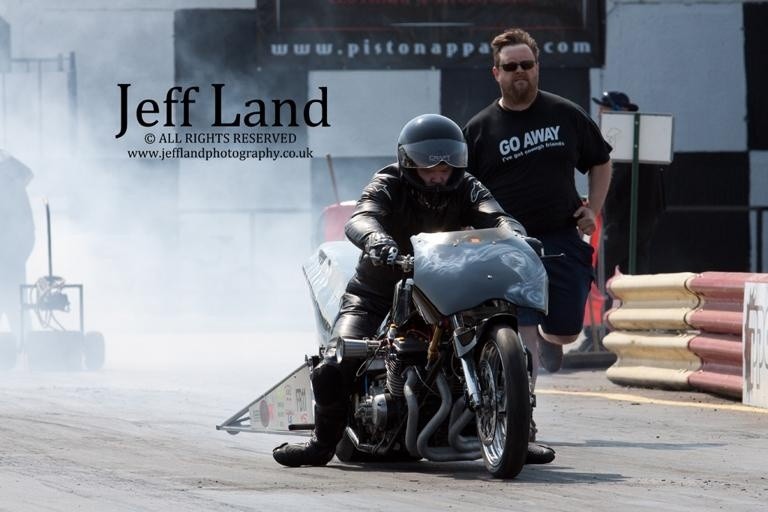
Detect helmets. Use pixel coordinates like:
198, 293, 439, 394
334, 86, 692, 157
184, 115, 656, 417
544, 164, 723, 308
397, 113, 470, 212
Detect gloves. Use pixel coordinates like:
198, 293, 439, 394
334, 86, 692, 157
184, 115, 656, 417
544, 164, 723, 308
365, 232, 399, 266
507, 231, 543, 248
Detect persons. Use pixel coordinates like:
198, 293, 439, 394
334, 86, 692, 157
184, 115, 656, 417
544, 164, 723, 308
456, 25, 617, 373
272, 113, 556, 474
0, 147, 39, 341
589, 88, 640, 314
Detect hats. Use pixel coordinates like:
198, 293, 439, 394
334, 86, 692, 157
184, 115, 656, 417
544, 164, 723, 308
592, 90, 639, 112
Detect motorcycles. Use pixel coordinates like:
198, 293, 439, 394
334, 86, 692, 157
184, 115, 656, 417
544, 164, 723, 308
290, 227, 566, 480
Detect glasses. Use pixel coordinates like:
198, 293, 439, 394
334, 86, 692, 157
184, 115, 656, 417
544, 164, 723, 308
495, 60, 535, 71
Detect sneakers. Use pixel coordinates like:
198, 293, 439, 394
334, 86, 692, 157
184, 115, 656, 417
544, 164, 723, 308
273, 442, 326, 467
524, 443, 556, 466
537, 325, 563, 372
